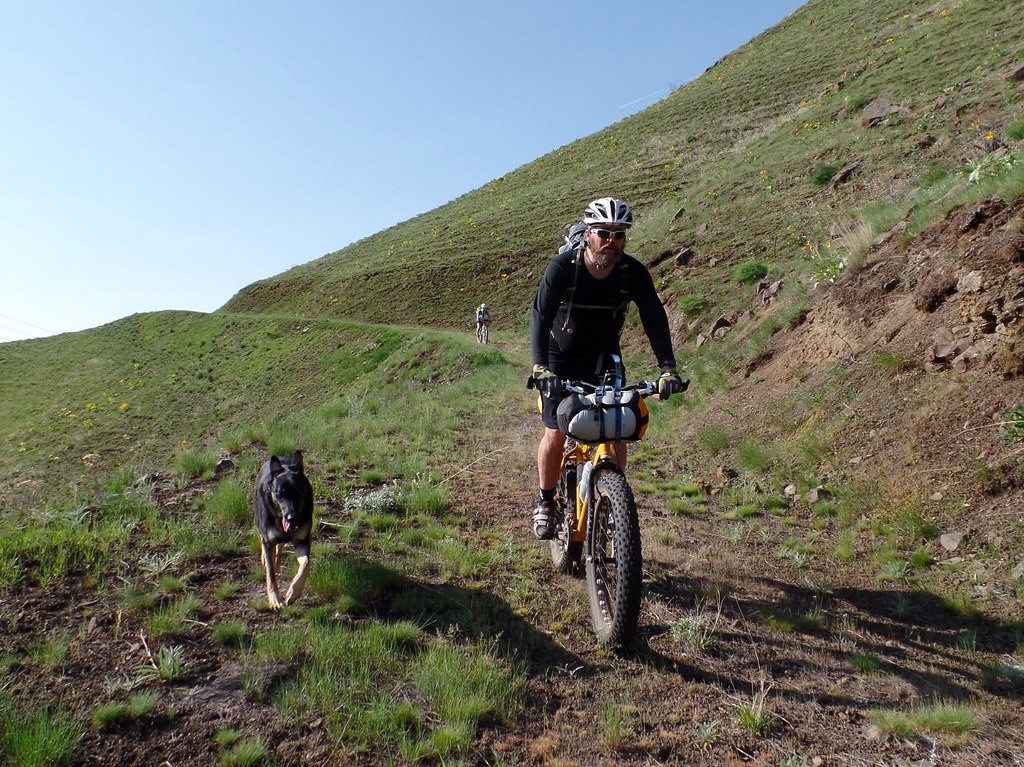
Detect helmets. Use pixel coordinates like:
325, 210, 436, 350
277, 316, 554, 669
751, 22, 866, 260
583, 197, 634, 229
481, 303, 486, 308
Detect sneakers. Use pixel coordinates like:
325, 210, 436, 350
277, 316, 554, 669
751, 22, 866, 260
532, 501, 554, 540
607, 514, 619, 541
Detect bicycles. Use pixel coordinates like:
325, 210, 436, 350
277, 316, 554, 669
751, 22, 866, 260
526, 368, 692, 654
476, 318, 496, 346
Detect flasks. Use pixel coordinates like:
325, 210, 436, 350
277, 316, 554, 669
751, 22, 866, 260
579, 459, 593, 503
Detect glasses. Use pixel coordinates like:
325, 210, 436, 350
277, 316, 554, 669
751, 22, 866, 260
589, 229, 626, 240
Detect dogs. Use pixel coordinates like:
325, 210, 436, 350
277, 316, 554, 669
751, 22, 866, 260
253, 448, 314, 611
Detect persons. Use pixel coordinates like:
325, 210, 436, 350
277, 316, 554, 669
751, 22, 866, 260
476, 303, 492, 337
530, 197, 683, 540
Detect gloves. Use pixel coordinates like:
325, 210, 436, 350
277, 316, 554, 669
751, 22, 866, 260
654, 366, 684, 400
531, 365, 562, 400
